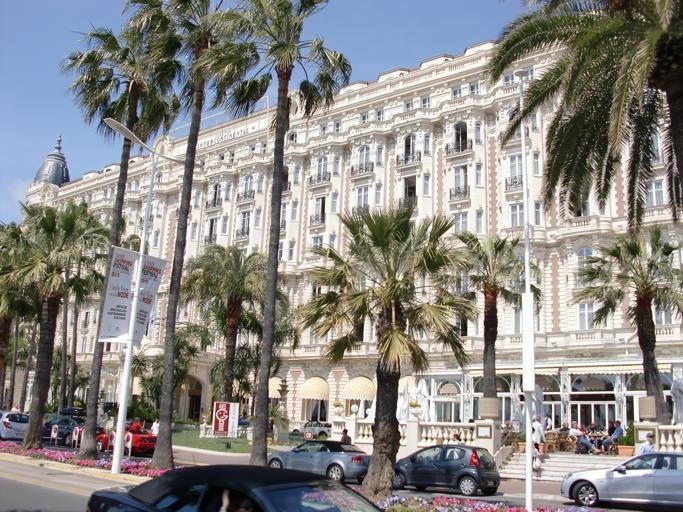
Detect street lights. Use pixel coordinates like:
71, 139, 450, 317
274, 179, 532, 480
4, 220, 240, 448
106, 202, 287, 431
101, 116, 204, 476
512, 67, 539, 512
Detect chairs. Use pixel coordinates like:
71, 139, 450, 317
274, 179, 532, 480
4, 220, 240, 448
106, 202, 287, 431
542, 428, 623, 454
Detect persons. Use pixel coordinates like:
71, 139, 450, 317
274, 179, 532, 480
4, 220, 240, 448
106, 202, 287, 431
530, 414, 624, 465
10, 402, 20, 412
340, 428, 350, 443
638, 432, 655, 468
149, 417, 159, 434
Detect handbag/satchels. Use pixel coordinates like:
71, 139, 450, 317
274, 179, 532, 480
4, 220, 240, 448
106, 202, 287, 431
532, 456, 542, 470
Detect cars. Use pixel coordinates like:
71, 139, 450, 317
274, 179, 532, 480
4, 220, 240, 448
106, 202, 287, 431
86, 463, 387, 512
267, 439, 371, 485
0, 406, 159, 458
390, 443, 501, 496
292, 418, 332, 438
559, 450, 683, 508
